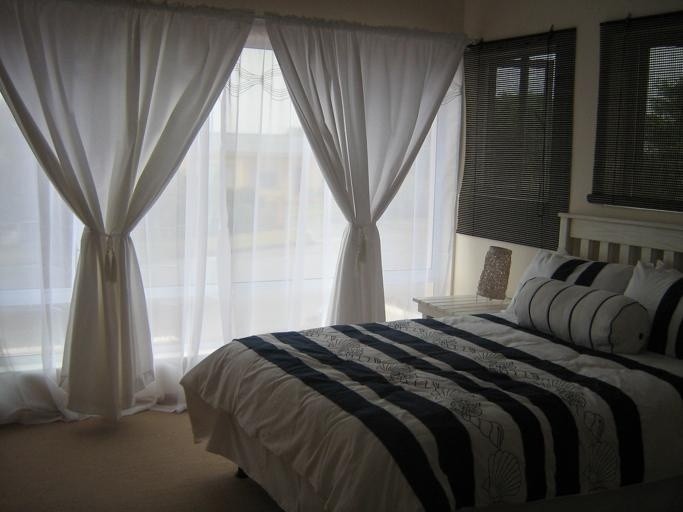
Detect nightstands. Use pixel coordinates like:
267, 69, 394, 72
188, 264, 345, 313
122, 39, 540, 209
413, 294, 508, 319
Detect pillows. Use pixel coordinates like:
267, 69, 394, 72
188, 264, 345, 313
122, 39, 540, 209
623, 261, 683, 360
513, 276, 650, 353
504, 249, 632, 319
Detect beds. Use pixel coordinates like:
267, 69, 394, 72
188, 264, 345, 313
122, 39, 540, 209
180, 212, 683, 511
